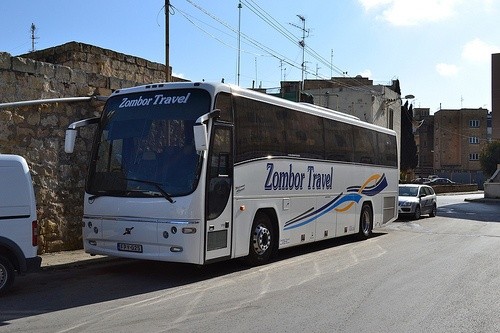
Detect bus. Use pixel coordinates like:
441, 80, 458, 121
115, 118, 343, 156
64, 78, 401, 267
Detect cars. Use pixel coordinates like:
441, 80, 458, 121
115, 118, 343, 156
399, 184, 437, 220
413, 177, 431, 183
0, 153, 45, 298
423, 178, 455, 185
